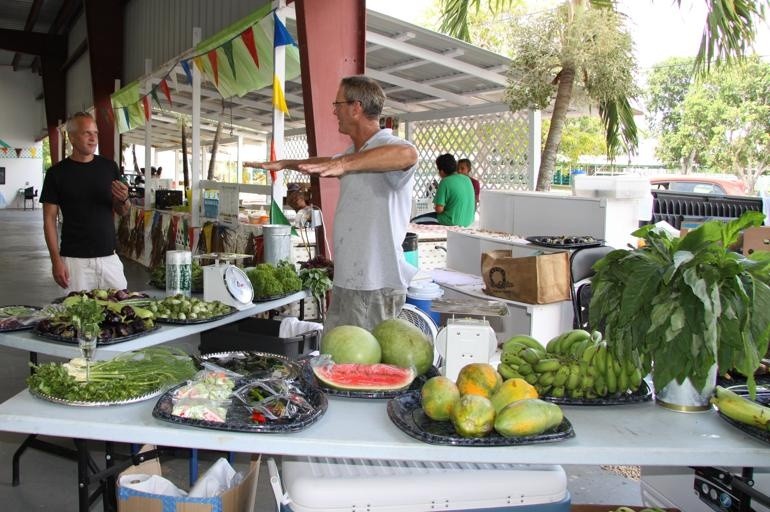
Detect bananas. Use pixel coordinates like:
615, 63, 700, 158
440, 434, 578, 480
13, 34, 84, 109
708, 397, 770, 430
497, 330, 642, 398
717, 385, 769, 411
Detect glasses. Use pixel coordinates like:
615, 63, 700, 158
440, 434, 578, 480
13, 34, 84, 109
332, 100, 362, 109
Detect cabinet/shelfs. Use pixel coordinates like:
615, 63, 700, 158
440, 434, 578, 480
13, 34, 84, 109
434, 183, 654, 348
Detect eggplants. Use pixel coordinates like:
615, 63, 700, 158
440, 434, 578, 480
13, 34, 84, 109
539, 235, 593, 244
69, 289, 149, 301
40, 306, 144, 340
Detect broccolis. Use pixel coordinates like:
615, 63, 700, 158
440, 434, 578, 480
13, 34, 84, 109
248, 263, 303, 297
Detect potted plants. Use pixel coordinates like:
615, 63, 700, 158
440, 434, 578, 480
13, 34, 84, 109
591, 209, 769, 411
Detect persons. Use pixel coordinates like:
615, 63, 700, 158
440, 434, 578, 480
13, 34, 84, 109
40, 112, 131, 295
457, 159, 480, 210
293, 192, 312, 227
242, 77, 420, 331
410, 153, 475, 229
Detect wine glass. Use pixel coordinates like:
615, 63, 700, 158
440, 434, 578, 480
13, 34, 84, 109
78, 325, 96, 383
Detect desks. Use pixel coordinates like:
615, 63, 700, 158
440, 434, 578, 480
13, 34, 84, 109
0, 353, 769, 511
0, 288, 306, 512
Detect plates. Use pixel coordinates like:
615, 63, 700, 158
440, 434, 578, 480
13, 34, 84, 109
728, 383, 768, 394
386, 391, 576, 446
62, 357, 199, 388
0, 288, 297, 347
301, 354, 443, 401
151, 376, 329, 435
198, 350, 301, 379
30, 368, 164, 407
711, 394, 770, 448
526, 236, 606, 249
499, 371, 653, 406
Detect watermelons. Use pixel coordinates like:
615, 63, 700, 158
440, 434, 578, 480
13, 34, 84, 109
372, 320, 433, 375
321, 326, 382, 363
314, 363, 417, 389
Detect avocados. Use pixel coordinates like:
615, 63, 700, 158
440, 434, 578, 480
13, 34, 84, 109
421, 364, 564, 437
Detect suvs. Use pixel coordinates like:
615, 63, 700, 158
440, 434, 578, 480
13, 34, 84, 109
646, 177, 750, 197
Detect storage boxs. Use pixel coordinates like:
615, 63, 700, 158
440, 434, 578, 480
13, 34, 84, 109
479, 249, 573, 305
268, 459, 571, 512
116, 446, 261, 512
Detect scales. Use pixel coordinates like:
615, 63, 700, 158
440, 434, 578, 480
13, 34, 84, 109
429, 299, 511, 384
193, 252, 257, 312
